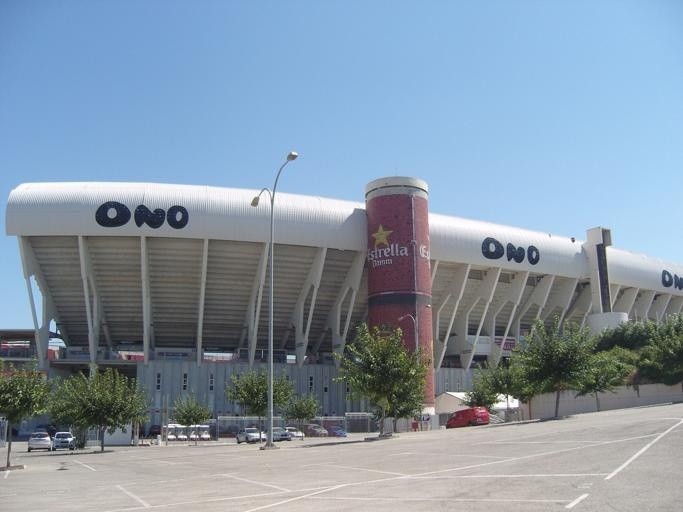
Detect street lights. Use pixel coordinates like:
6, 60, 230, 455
249, 148, 298, 451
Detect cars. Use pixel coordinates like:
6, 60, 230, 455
236, 427, 268, 445
283, 427, 305, 440
326, 425, 347, 437
27, 431, 52, 452
51, 431, 77, 452
262, 426, 292, 442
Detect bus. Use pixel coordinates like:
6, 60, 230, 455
189, 424, 211, 441
163, 423, 188, 442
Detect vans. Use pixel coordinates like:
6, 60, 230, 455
300, 424, 328, 437
446, 405, 490, 429
